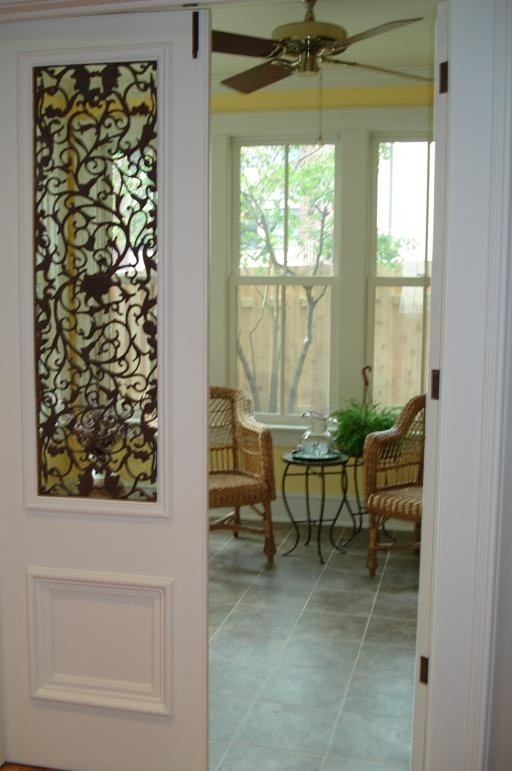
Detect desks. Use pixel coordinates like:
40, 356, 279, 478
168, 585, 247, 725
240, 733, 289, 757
282, 453, 351, 565
327, 449, 404, 547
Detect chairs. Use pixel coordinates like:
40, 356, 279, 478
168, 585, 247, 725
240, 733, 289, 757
359, 394, 428, 581
207, 384, 279, 570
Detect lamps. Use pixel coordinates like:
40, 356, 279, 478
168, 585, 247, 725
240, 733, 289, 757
283, 42, 324, 80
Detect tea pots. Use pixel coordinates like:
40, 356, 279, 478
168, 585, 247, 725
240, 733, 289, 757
299, 410, 341, 459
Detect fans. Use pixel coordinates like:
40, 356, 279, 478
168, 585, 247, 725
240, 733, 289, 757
211, 1, 434, 97
